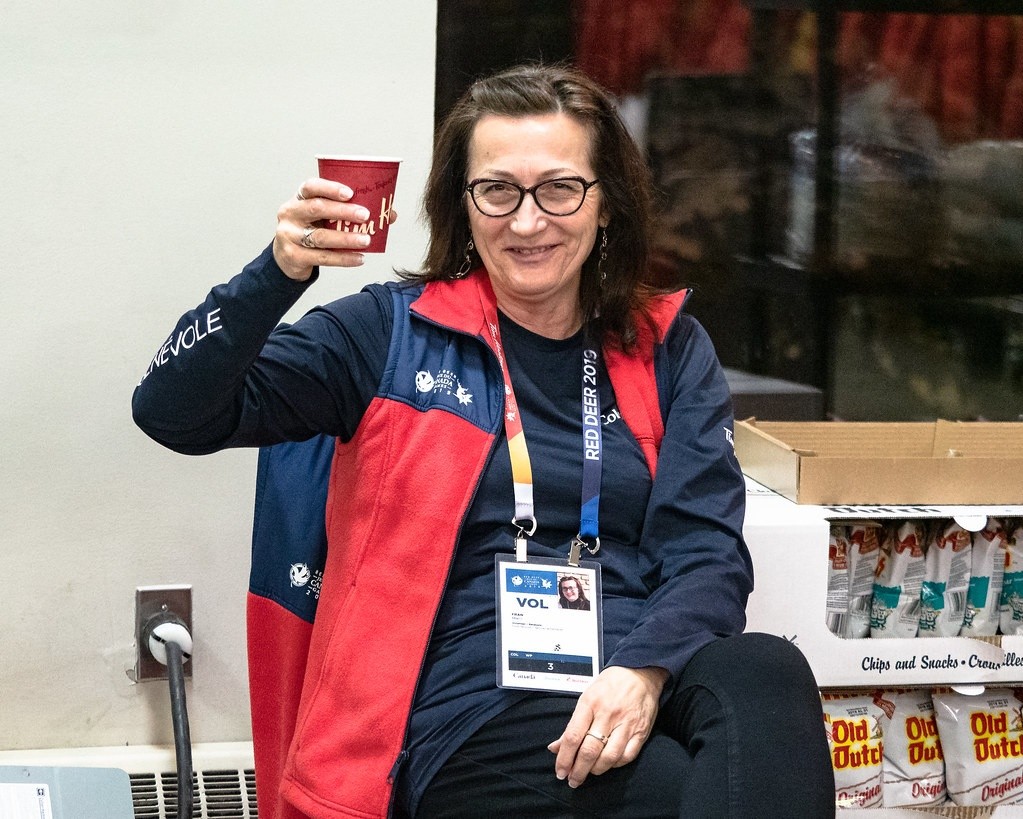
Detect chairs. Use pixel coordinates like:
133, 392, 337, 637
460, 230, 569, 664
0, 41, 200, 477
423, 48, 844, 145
247, 436, 335, 818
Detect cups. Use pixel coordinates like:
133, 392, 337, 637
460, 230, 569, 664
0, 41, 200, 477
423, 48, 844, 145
314, 154, 403, 253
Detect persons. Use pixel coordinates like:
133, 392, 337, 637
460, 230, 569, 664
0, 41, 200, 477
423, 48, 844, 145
557, 576, 590, 611
130, 61, 835, 818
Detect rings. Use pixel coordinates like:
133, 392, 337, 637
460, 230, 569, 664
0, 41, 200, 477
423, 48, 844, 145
302, 226, 321, 247
585, 730, 612, 743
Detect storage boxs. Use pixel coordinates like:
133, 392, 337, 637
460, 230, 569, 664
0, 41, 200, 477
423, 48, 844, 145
744, 473, 1023, 688
734, 417, 1023, 507
820, 682, 1023, 819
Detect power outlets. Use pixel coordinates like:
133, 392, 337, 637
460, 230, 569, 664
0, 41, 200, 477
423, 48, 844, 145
135, 585, 194, 683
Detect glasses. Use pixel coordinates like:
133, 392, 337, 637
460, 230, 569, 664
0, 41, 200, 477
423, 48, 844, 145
562, 586, 577, 592
466, 175, 599, 217
